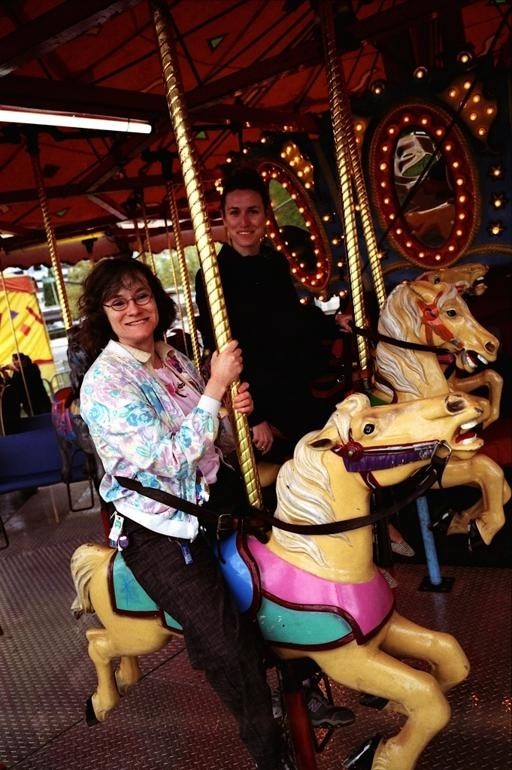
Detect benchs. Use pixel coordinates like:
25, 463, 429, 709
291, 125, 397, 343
2, 414, 79, 492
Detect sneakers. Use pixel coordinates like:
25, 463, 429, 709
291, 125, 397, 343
378, 540, 415, 589
271, 687, 356, 730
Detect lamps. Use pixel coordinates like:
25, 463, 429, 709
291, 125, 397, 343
0, 103, 152, 136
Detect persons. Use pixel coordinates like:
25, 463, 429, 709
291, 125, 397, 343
194, 172, 418, 591
12, 352, 52, 417
73, 253, 355, 770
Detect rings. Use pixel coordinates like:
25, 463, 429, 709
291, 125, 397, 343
261, 446, 267, 451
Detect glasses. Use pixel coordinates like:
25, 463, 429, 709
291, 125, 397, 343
103, 288, 154, 311
